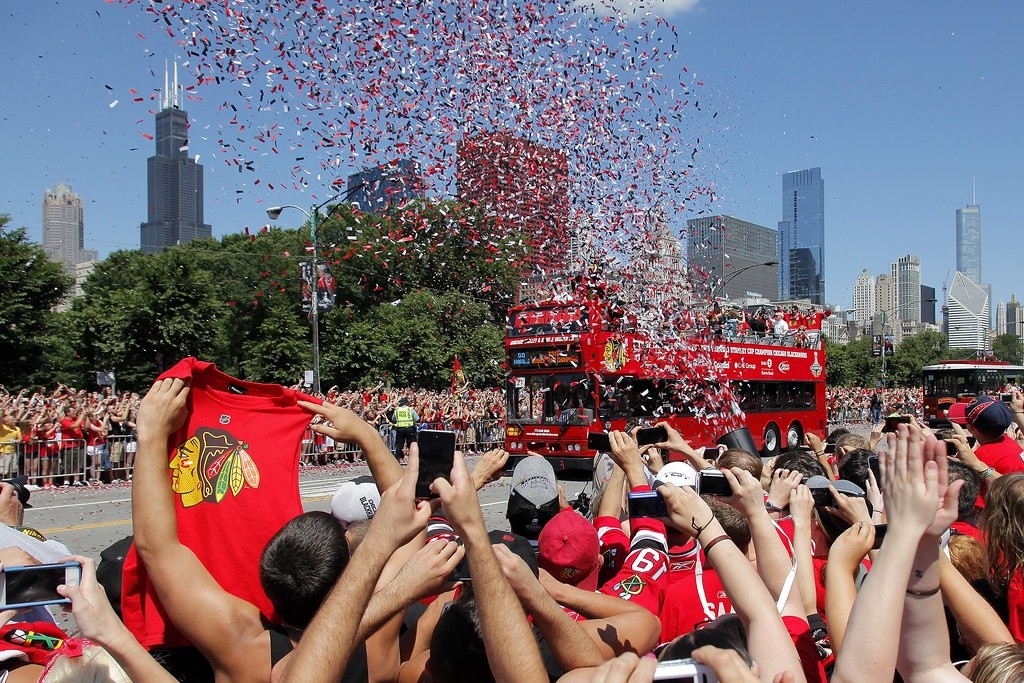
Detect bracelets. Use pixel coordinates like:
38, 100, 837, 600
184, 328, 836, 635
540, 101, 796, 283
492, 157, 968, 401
703, 535, 731, 556
979, 467, 995, 480
978, 467, 990, 473
814, 450, 823, 454
816, 453, 825, 457
692, 513, 714, 539
906, 583, 941, 596
873, 510, 882, 514
1011, 410, 1024, 413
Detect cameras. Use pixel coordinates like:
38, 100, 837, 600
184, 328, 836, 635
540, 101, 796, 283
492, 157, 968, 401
653, 657, 718, 683
0, 475, 33, 508
628, 490, 669, 518
700, 470, 740, 496
999, 393, 1018, 403
798, 477, 809, 484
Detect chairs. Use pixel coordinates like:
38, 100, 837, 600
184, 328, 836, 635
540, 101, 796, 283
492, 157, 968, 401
732, 328, 821, 349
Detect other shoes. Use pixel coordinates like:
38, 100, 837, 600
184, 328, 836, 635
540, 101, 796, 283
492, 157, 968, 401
26, 476, 132, 491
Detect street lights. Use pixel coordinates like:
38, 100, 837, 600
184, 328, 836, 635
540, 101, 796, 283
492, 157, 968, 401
670, 261, 779, 301
955, 321, 1024, 360
265, 175, 401, 395
846, 299, 938, 388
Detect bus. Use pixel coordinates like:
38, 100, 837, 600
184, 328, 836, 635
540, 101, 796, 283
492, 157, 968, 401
504, 289, 827, 469
921, 360, 1024, 426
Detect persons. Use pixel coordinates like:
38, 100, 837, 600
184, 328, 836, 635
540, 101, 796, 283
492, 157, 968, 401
0, 378, 1024, 683
615, 300, 833, 348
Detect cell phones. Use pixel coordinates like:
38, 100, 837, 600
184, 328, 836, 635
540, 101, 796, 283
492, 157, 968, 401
448, 552, 472, 581
859, 523, 887, 550
0, 561, 82, 611
415, 429, 456, 499
636, 426, 667, 445
946, 442, 956, 455
703, 448, 719, 459
882, 416, 910, 433
825, 444, 836, 453
868, 455, 881, 491
588, 432, 612, 452
809, 487, 832, 507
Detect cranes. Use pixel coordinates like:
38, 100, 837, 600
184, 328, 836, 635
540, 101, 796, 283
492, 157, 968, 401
942, 268, 950, 332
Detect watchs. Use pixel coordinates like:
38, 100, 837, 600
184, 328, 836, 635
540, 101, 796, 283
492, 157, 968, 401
764, 501, 782, 512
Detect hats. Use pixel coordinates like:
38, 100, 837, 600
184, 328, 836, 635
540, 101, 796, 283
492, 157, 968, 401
507, 456, 560, 535
398, 398, 409, 404
331, 476, 381, 521
948, 399, 1013, 437
775, 312, 784, 318
805, 475, 874, 542
656, 462, 698, 487
538, 510, 598, 593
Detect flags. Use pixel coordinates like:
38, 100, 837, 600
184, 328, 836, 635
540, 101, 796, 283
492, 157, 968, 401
452, 358, 465, 389
